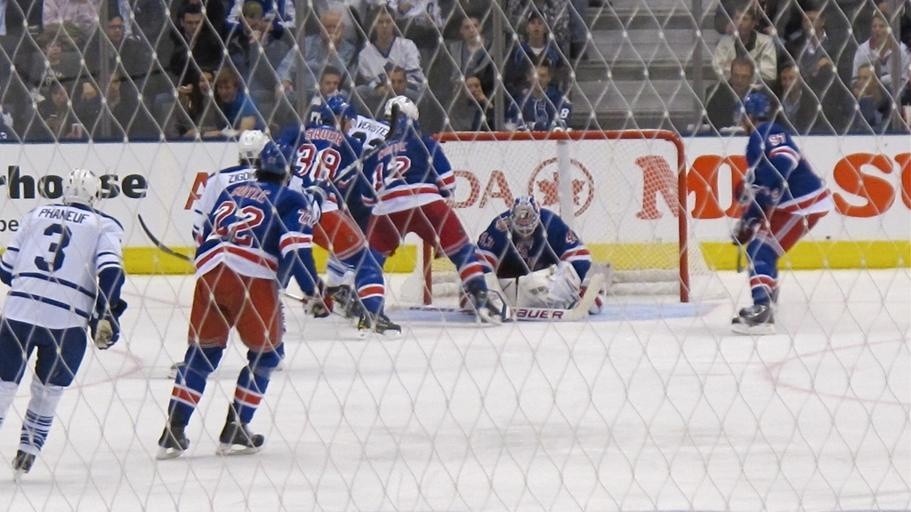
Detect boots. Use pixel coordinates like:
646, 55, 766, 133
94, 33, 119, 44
220, 367, 269, 448
157, 368, 206, 449
459, 261, 509, 323
731, 258, 777, 325
12, 404, 55, 473
323, 238, 401, 333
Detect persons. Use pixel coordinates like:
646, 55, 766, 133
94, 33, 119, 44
0, 0, 575, 141
703, 0, 911, 136
193, 95, 504, 334
458, 195, 605, 321
0, 167, 127, 474
158, 141, 333, 451
731, 93, 833, 326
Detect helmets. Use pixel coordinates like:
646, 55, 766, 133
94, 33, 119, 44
238, 130, 271, 161
508, 196, 543, 234
62, 170, 103, 209
381, 97, 419, 123
320, 93, 358, 132
259, 139, 296, 174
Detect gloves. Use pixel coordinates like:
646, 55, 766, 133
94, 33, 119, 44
730, 215, 757, 246
91, 305, 120, 351
740, 168, 760, 205
304, 280, 333, 319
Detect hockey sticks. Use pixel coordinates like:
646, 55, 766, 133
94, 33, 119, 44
387, 274, 604, 322
138, 215, 350, 320
736, 183, 748, 272
